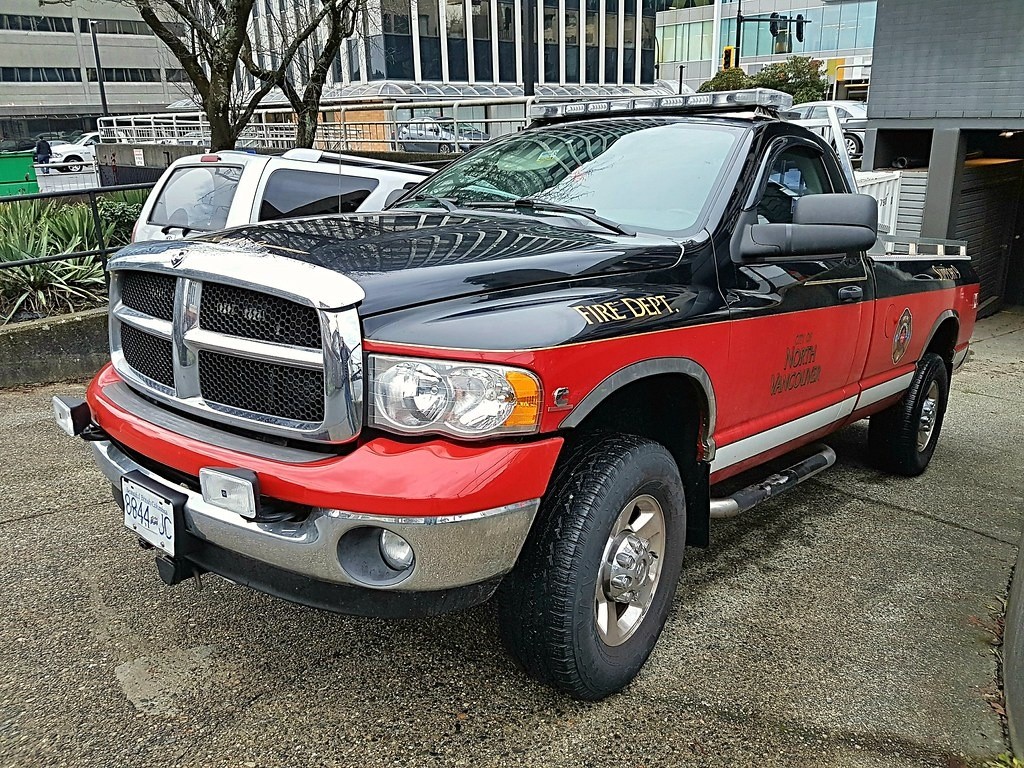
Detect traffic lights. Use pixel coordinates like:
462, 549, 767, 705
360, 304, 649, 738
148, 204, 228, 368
723, 47, 734, 70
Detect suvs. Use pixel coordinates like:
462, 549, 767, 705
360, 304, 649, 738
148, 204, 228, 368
128, 146, 441, 245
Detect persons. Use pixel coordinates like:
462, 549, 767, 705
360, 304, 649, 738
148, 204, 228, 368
36, 135, 52, 174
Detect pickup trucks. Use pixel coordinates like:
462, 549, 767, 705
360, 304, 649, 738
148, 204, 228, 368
48, 131, 171, 174
53, 86, 977, 702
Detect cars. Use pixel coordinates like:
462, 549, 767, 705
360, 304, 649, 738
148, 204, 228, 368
390, 115, 492, 152
32, 132, 64, 141
31, 141, 70, 161
0, 137, 36, 152
785, 99, 868, 169
62, 130, 83, 142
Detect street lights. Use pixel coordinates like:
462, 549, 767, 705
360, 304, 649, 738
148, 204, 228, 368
89, 20, 109, 115
